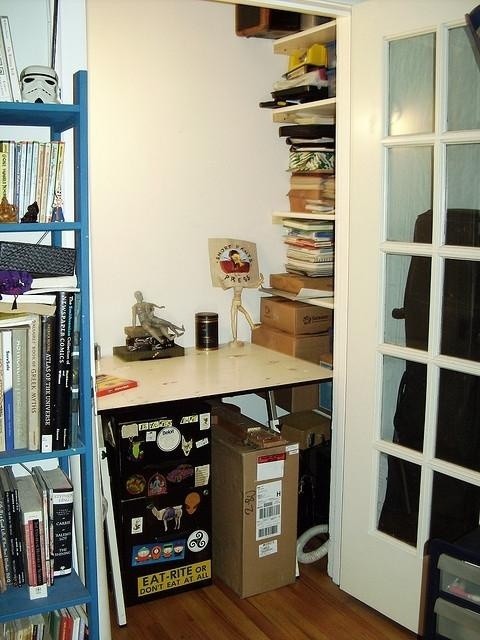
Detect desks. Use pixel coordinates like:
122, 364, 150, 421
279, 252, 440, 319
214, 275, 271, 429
92, 338, 333, 627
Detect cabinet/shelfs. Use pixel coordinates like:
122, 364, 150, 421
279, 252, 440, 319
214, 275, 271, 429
261, 17, 337, 313
0, 71, 101, 640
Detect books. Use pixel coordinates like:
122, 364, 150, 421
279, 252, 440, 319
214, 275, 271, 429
1, 141, 65, 224
0, 16, 23, 103
279, 116, 335, 278
3, 607, 88, 640
285, 63, 325, 80
1, 241, 81, 453
96, 374, 137, 397
0, 466, 74, 600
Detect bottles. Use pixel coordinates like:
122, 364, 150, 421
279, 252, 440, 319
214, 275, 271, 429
195, 312, 218, 351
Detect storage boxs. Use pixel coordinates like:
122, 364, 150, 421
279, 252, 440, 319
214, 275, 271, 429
285, 189, 321, 213
249, 328, 329, 359
198, 403, 301, 601
276, 410, 330, 449
259, 295, 332, 335
105, 403, 214, 606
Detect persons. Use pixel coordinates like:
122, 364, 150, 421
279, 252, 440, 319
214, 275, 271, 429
132, 291, 185, 345
220, 273, 264, 344
